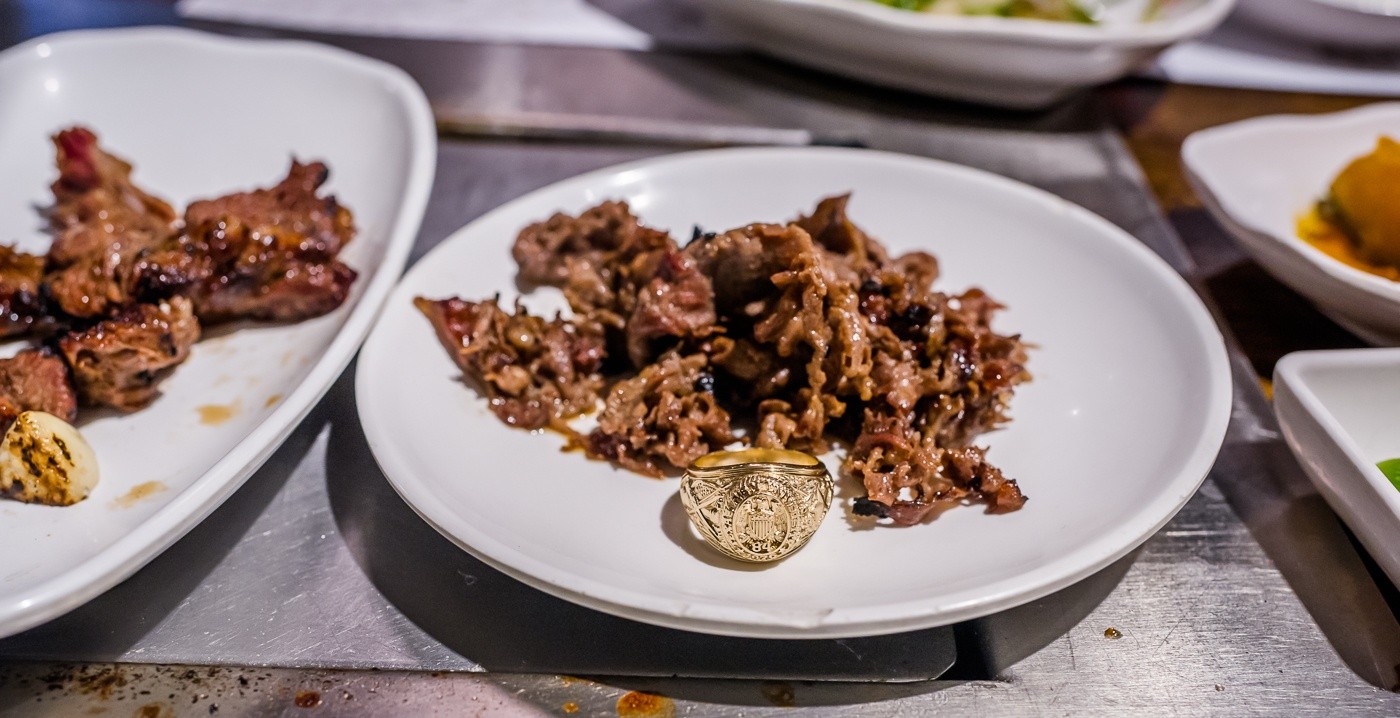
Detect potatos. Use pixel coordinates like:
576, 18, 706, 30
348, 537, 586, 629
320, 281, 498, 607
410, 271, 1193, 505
0, 411, 100, 505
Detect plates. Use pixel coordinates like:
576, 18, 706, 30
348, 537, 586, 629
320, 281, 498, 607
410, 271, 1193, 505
353, 145, 1234, 639
1273, 345, 1400, 603
0, 25, 440, 642
1180, 102, 1399, 349
606, 0, 1400, 109
